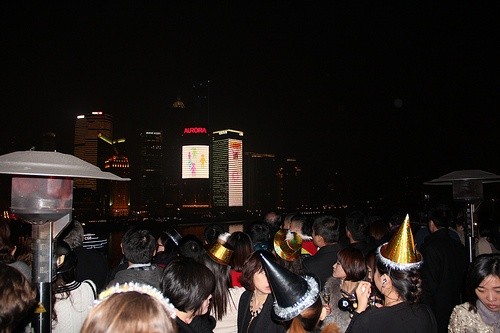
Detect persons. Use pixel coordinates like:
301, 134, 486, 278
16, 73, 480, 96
63, 219, 106, 292
420, 203, 466, 333
106, 226, 164, 291
0, 206, 500, 333
321, 246, 371, 331
347, 211, 376, 256
237, 249, 283, 333
304, 217, 343, 282
449, 252, 500, 333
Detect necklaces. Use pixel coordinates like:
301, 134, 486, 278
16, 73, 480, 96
250, 299, 264, 316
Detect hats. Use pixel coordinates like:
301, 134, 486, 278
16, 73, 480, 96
203, 241, 235, 266
260, 253, 319, 321
376, 213, 424, 271
163, 229, 184, 247
55, 239, 78, 274
274, 228, 303, 261
217, 232, 231, 244
